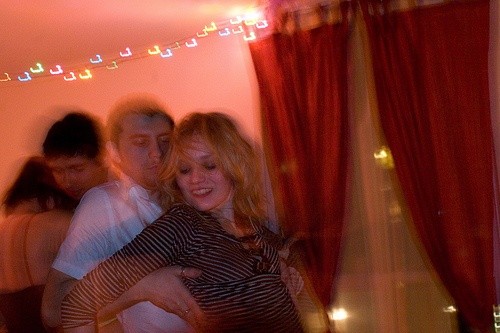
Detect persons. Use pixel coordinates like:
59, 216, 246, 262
41, 112, 108, 203
0, 154, 80, 333
58, 112, 330, 333
40, 107, 305, 333
